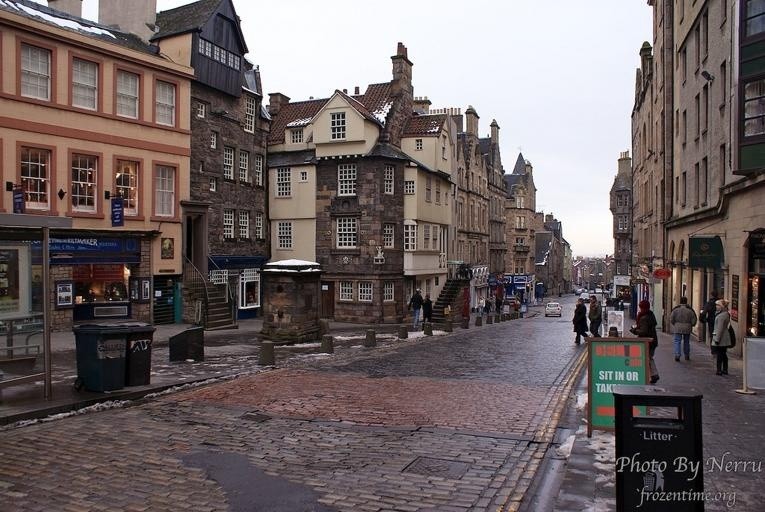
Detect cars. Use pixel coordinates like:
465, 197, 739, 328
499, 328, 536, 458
575, 286, 604, 295
579, 293, 590, 303
545, 302, 562, 317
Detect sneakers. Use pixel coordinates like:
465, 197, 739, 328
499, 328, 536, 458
649, 375, 660, 384
716, 369, 729, 376
675, 356, 691, 362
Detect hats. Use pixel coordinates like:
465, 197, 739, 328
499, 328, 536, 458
716, 299, 730, 309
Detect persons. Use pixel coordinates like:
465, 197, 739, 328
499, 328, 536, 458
588, 295, 601, 338
609, 314, 622, 331
710, 299, 732, 375
629, 299, 660, 384
669, 296, 697, 361
422, 294, 432, 330
573, 298, 588, 344
476, 294, 510, 318
699, 287, 718, 341
408, 289, 423, 329
609, 311, 616, 324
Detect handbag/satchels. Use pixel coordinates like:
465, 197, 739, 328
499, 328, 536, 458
727, 325, 737, 349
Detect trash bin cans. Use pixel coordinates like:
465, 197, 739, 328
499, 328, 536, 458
612, 385, 704, 512
71, 321, 157, 393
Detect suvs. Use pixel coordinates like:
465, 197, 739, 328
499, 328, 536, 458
602, 289, 623, 298
